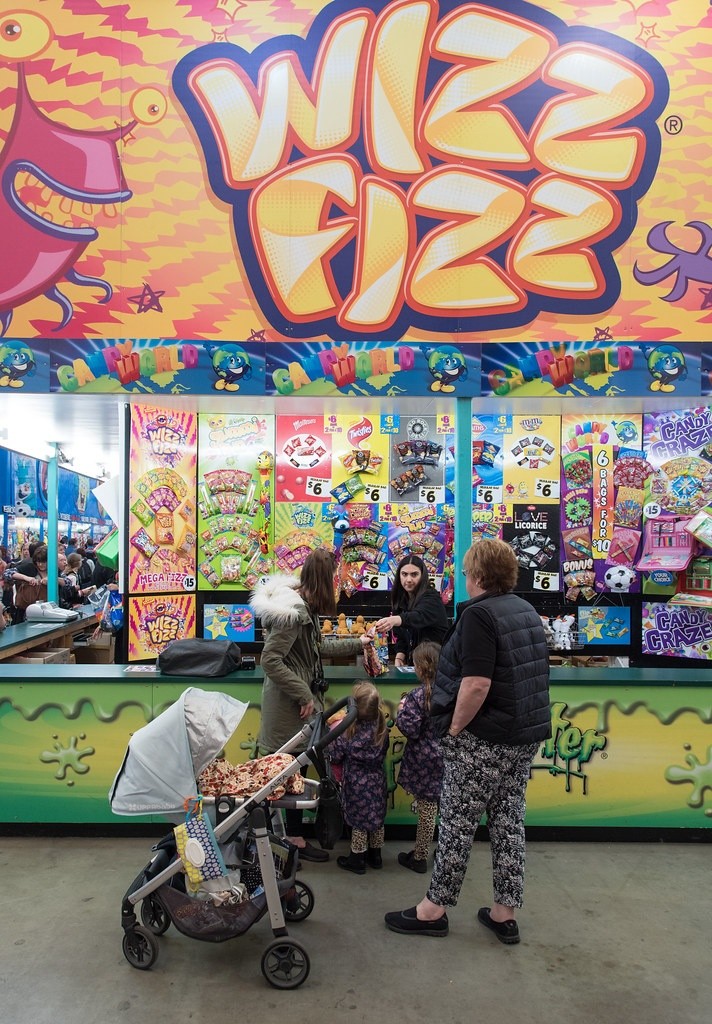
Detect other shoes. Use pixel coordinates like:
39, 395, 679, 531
477, 907, 520, 944
296, 841, 330, 863
295, 861, 302, 871
384, 906, 449, 937
398, 850, 427, 873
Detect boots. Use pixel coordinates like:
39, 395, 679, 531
337, 851, 366, 875
365, 847, 383, 870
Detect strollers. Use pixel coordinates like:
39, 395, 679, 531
109, 686, 360, 992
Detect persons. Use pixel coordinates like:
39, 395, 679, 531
323, 642, 444, 875
383, 538, 552, 947
372, 554, 451, 670
0, 524, 115, 634
257, 550, 377, 863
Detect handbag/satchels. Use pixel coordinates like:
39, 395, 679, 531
309, 751, 343, 850
88, 585, 123, 632
15, 582, 47, 608
159, 638, 242, 678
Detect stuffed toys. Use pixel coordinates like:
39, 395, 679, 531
538, 613, 576, 651
321, 612, 377, 634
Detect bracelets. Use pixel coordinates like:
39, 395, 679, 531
398, 702, 404, 707
449, 725, 463, 733
394, 657, 404, 662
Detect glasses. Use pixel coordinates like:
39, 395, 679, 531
462, 568, 469, 576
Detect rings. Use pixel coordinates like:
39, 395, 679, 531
390, 627, 392, 629
